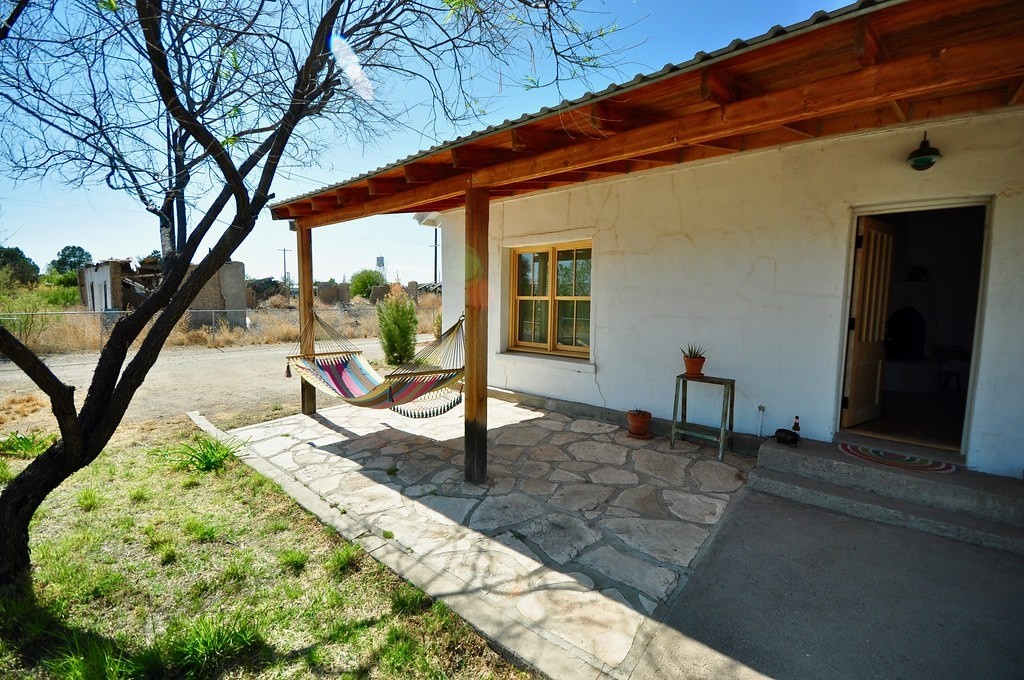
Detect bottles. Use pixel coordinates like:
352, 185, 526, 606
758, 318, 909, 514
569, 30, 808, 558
792, 416, 800, 435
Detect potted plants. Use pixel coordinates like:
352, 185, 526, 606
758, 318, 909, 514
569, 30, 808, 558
680, 342, 708, 376
623, 405, 655, 439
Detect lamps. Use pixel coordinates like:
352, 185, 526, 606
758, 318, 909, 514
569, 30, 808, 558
906, 131, 943, 172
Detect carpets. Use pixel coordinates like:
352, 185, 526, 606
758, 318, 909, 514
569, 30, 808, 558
838, 444, 957, 474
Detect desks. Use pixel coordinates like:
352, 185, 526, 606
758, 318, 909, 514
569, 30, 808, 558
671, 375, 736, 461
